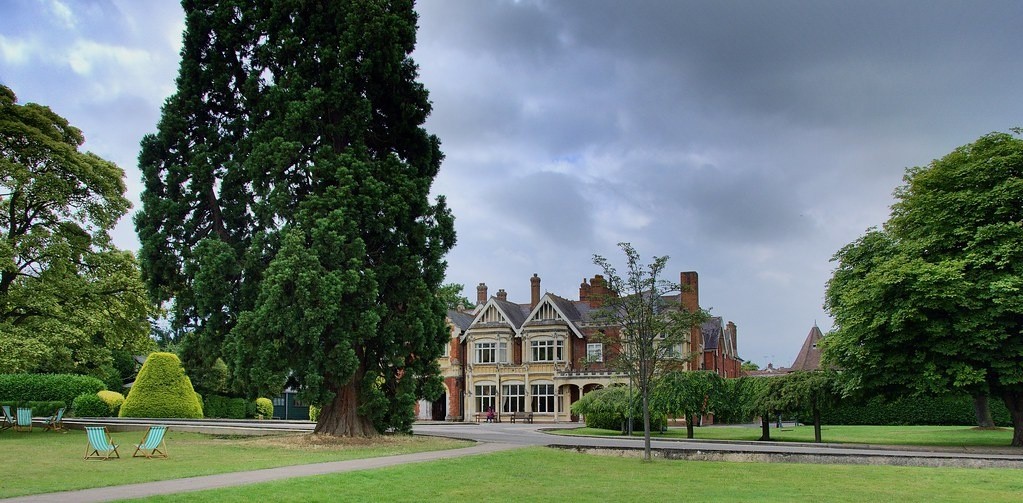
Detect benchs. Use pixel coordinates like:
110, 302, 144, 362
511, 412, 534, 424
476, 412, 498, 422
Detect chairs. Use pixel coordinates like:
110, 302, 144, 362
1, 406, 17, 431
132, 426, 169, 458
83, 426, 120, 460
41, 408, 65, 432
17, 406, 33, 432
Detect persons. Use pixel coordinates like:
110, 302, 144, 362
484, 406, 494, 423
